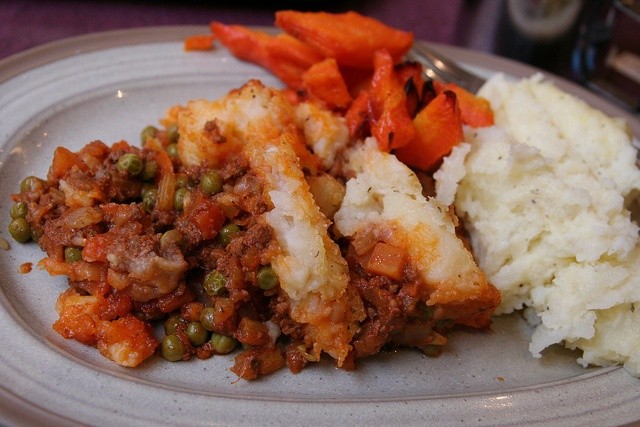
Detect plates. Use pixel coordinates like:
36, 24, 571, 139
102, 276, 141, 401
1, 26, 640, 427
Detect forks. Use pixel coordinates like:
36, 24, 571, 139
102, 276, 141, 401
400, 33, 486, 96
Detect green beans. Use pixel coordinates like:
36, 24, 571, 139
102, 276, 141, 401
8, 124, 277, 363
